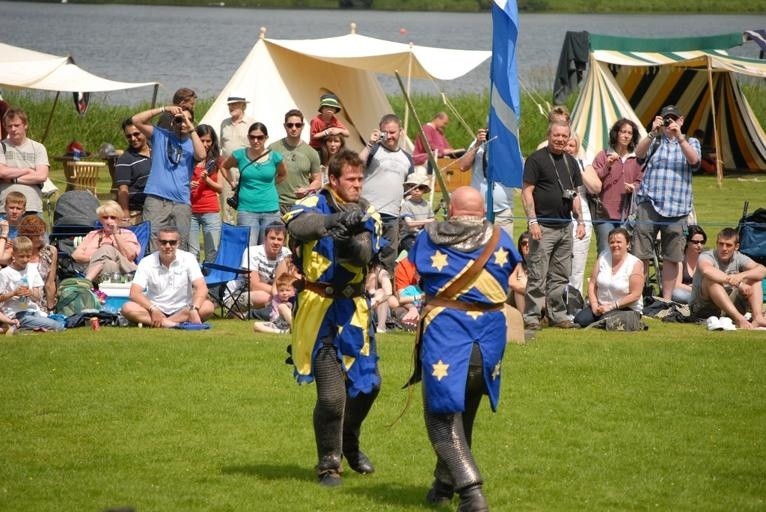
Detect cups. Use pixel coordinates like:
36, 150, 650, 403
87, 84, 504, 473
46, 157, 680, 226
124, 272, 133, 283
111, 272, 121, 283
89, 316, 100, 330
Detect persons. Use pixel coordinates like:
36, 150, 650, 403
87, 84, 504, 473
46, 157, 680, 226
457, 115, 515, 239
508, 105, 766, 331
406, 185, 526, 511
410, 111, 453, 158
280, 147, 393, 487
0, 87, 444, 338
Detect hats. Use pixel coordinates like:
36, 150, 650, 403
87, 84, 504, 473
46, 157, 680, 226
404, 173, 431, 193
318, 95, 342, 113
662, 105, 680, 119
226, 95, 250, 104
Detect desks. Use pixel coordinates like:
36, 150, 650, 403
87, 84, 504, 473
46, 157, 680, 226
63, 160, 105, 196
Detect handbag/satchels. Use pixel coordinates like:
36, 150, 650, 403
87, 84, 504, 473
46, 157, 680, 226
605, 311, 640, 331
586, 196, 609, 224
57, 278, 95, 317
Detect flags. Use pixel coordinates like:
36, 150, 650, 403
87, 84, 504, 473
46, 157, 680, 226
486, 0, 526, 189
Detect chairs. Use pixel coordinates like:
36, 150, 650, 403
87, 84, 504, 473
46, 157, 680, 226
78, 223, 150, 287
201, 220, 251, 319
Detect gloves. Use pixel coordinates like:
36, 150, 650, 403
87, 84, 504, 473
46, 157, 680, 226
334, 228, 362, 258
340, 210, 365, 227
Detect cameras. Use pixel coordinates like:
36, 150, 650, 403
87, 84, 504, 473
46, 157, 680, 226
661, 119, 671, 128
225, 196, 239, 210
562, 188, 578, 200
172, 116, 183, 126
379, 132, 388, 142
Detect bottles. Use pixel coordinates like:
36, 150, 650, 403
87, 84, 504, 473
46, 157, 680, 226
19, 279, 29, 309
6, 324, 17, 335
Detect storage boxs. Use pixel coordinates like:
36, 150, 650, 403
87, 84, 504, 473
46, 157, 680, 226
434, 160, 472, 191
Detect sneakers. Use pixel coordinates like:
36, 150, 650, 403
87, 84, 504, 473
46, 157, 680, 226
556, 320, 572, 328
720, 317, 736, 331
456, 495, 488, 511
528, 323, 538, 330
317, 469, 342, 486
345, 449, 374, 474
427, 488, 452, 508
707, 316, 723, 330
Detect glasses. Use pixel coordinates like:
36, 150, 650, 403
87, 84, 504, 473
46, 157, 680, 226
127, 133, 139, 138
286, 123, 303, 128
520, 240, 528, 245
689, 239, 706, 245
159, 239, 178, 245
249, 135, 264, 140
